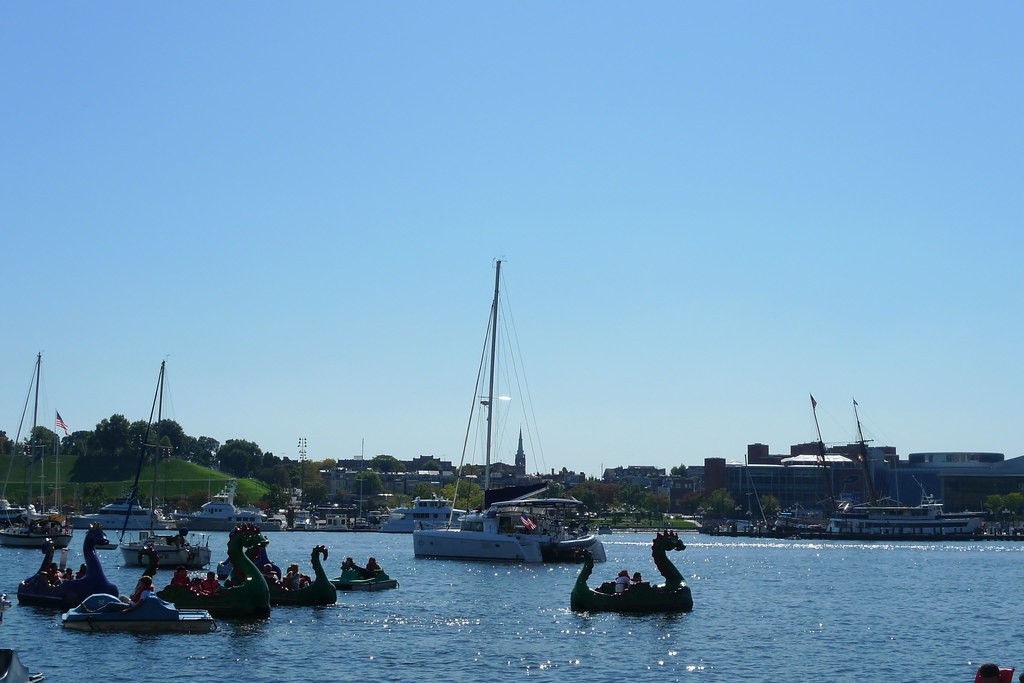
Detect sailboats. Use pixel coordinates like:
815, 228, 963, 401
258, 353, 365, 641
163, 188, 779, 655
412, 257, 613, 565
1, 351, 77, 550
67, 359, 175, 528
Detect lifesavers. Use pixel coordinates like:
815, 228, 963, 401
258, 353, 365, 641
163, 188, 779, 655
937, 511, 941, 515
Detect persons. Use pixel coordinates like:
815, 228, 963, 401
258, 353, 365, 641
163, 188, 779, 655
980, 664, 1001, 683
49, 563, 87, 586
262, 564, 311, 590
367, 558, 376, 570
581, 522, 589, 535
616, 570, 642, 593
171, 566, 221, 596
119, 576, 155, 602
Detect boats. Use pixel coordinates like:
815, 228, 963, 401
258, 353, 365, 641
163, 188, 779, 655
169, 476, 286, 535
119, 530, 211, 567
695, 397, 989, 543
0, 648, 45, 683
290, 509, 371, 532
132, 522, 274, 622
213, 536, 338, 607
60, 545, 220, 636
377, 492, 469, 536
567, 527, 698, 617
12, 521, 121, 611
325, 556, 402, 592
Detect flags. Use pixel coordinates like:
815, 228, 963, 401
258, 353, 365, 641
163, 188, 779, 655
519, 515, 536, 530
811, 395, 816, 408
854, 400, 858, 405
57, 412, 68, 429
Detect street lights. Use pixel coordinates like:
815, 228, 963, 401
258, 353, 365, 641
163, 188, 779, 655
297, 436, 308, 510
